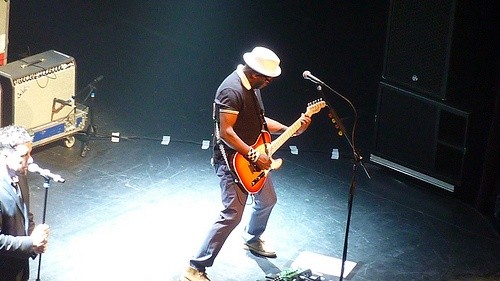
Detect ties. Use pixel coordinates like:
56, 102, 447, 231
10, 179, 24, 211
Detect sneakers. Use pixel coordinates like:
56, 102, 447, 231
241, 236, 276, 258
179, 262, 213, 281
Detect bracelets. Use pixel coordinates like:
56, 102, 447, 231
246, 146, 260, 162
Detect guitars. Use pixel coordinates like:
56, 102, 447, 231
229, 98, 326, 194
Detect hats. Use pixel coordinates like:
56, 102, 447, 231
243, 45, 283, 78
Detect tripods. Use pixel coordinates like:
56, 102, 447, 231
74, 87, 112, 157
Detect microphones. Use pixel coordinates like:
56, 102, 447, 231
303, 70, 327, 87
72, 75, 105, 99
28, 163, 66, 183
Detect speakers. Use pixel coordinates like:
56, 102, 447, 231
0, 49, 77, 134
381, 0, 457, 101
371, 75, 481, 196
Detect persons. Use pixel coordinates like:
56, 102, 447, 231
182, 46, 311, 281
0, 125, 50, 281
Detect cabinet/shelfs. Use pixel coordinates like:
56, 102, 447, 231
371, 81, 473, 187
